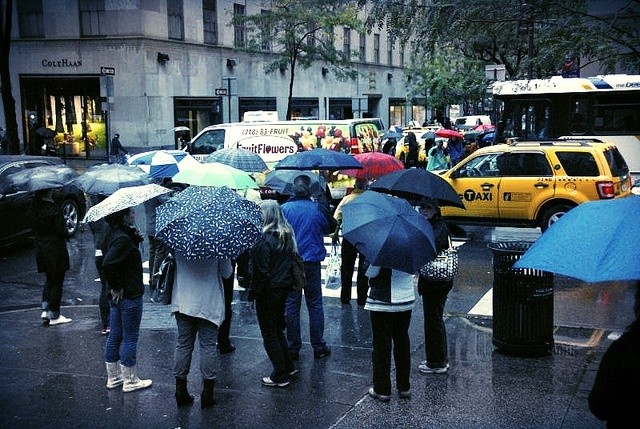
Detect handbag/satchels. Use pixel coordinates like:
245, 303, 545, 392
238, 248, 252, 289
420, 221, 460, 281
150, 256, 175, 305
291, 251, 307, 290
325, 244, 342, 290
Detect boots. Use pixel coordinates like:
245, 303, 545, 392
105, 360, 123, 389
200, 378, 216, 409
120, 364, 152, 393
174, 375, 194, 409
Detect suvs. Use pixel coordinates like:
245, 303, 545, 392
413, 139, 632, 235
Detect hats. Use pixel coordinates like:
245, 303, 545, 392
114, 133, 120, 138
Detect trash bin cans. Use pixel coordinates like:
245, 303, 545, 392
487, 241, 554, 355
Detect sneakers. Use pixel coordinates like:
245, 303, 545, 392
398, 390, 412, 399
419, 365, 447, 374
314, 346, 331, 359
106, 327, 111, 333
48, 315, 73, 325
368, 387, 391, 402
420, 360, 450, 368
288, 350, 299, 361
41, 311, 48, 319
219, 343, 235, 355
289, 369, 299, 380
102, 329, 106, 335
261, 376, 290, 386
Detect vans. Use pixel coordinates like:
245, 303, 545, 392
182, 121, 383, 172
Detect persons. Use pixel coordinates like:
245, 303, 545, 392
426, 139, 448, 170
178, 131, 190, 150
170, 250, 232, 410
363, 264, 417, 402
109, 133, 128, 164
587, 287, 639, 428
333, 178, 371, 305
0, 127, 9, 152
280, 175, 338, 360
44, 138, 55, 157
248, 199, 299, 387
85, 195, 112, 335
143, 198, 160, 290
418, 197, 458, 373
383, 138, 397, 157
425, 138, 436, 157
155, 177, 190, 196
29, 187, 73, 325
446, 136, 465, 167
101, 207, 153, 392
234, 174, 261, 203
406, 132, 419, 168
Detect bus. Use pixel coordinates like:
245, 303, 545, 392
492, 74, 639, 173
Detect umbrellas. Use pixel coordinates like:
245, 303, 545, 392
273, 147, 364, 171
154, 186, 264, 263
126, 150, 199, 179
368, 166, 466, 210
0, 164, 79, 192
381, 131, 402, 139
172, 126, 190, 131
463, 123, 497, 144
340, 190, 437, 274
512, 195, 639, 285
421, 132, 436, 138
75, 163, 150, 197
339, 150, 405, 180
82, 182, 172, 223
36, 128, 58, 139
171, 162, 259, 190
435, 129, 463, 139
264, 169, 328, 197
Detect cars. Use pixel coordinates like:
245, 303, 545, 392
0, 154, 86, 245
402, 124, 444, 135
395, 133, 429, 164
452, 114, 491, 132
